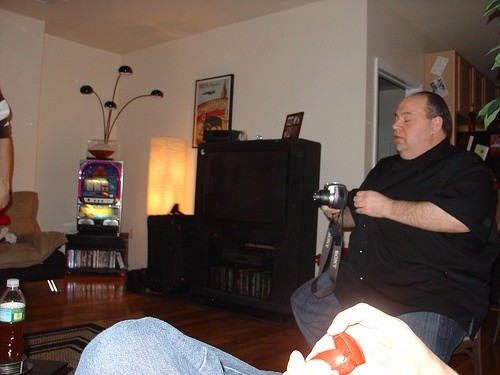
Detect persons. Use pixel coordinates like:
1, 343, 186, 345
0, 90, 13, 210
76, 302, 458, 375
290, 91, 499, 364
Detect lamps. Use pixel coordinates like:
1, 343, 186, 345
77, 65, 165, 157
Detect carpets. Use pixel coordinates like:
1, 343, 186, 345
23, 321, 108, 375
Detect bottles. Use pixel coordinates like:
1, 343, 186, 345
0, 277, 25, 365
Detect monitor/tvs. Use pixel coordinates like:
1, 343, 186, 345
204, 149, 289, 231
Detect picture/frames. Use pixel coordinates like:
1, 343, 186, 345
192, 73, 234, 149
282, 111, 304, 139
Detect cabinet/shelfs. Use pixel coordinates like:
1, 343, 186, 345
424, 50, 500, 153
65, 233, 127, 275
148, 138, 321, 326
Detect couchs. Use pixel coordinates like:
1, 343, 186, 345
0, 191, 68, 284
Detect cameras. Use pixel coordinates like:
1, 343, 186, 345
312, 184, 348, 209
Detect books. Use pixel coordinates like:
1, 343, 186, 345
210, 265, 271, 298
65, 250, 124, 270
245, 243, 274, 250
